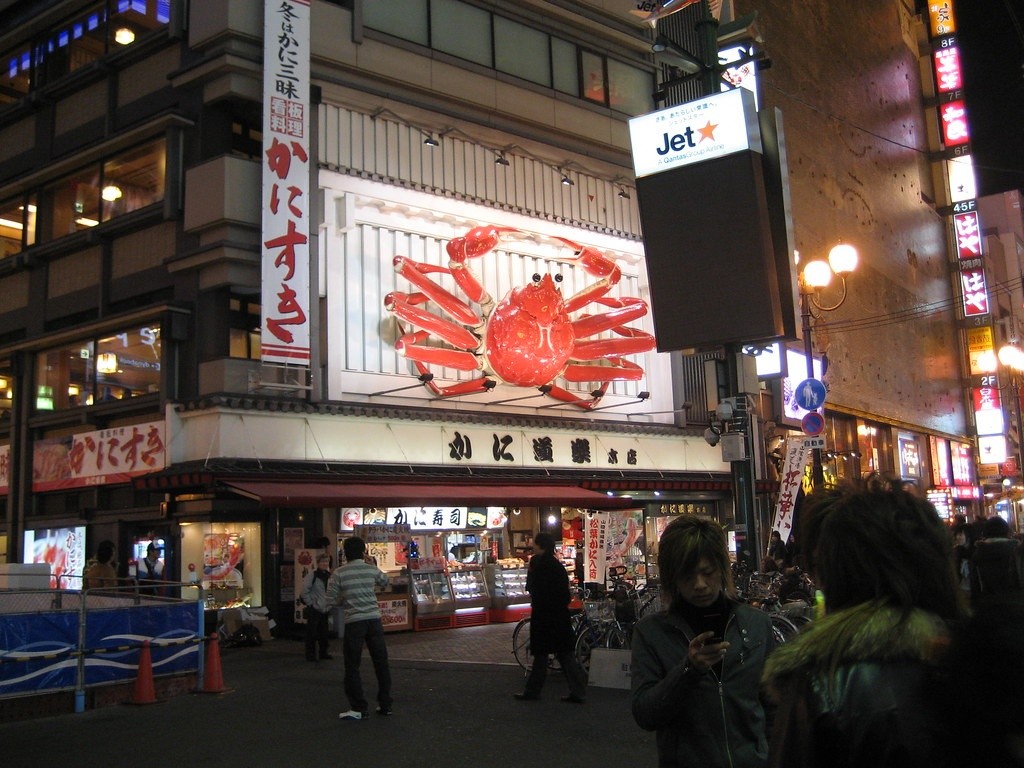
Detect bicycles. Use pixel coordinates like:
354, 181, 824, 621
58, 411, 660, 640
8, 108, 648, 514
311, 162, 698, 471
512, 566, 817, 678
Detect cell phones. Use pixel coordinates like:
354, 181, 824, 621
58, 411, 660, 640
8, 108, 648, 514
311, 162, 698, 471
704, 614, 724, 646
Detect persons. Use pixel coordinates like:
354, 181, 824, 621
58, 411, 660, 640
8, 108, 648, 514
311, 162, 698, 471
768, 531, 784, 563
760, 480, 1024, 768
302, 552, 335, 661
631, 516, 781, 768
82, 540, 166, 597
319, 536, 394, 719
784, 531, 800, 564
951, 514, 1024, 613
512, 532, 585, 705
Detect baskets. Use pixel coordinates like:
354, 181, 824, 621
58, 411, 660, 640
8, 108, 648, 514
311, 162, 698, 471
584, 600, 615, 621
634, 588, 669, 620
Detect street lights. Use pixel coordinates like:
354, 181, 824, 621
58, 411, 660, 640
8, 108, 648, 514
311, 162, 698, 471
796, 245, 859, 488
998, 345, 1024, 474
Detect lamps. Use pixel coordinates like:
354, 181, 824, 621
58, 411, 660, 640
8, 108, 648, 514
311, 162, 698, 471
536, 390, 603, 409
769, 434, 784, 442
369, 374, 434, 397
583, 391, 650, 412
486, 385, 552, 405
821, 450, 862, 460
439, 127, 511, 164
372, 109, 439, 147
652, 35, 665, 51
501, 145, 574, 185
428, 380, 496, 400
627, 402, 693, 416
557, 160, 631, 200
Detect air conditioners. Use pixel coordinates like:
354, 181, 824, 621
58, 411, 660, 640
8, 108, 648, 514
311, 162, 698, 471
721, 397, 747, 410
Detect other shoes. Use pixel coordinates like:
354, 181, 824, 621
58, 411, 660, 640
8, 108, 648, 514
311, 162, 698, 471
376, 706, 393, 715
307, 656, 320, 663
339, 710, 363, 720
561, 694, 581, 703
514, 693, 539, 700
319, 654, 334, 660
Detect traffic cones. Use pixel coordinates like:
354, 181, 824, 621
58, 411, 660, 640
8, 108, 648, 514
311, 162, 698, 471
121, 640, 168, 707
192, 634, 235, 694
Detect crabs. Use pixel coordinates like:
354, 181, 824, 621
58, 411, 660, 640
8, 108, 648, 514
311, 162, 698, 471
382, 224, 656, 410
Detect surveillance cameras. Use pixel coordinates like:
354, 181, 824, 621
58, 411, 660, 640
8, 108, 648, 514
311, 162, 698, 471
704, 427, 720, 446
716, 402, 732, 422
